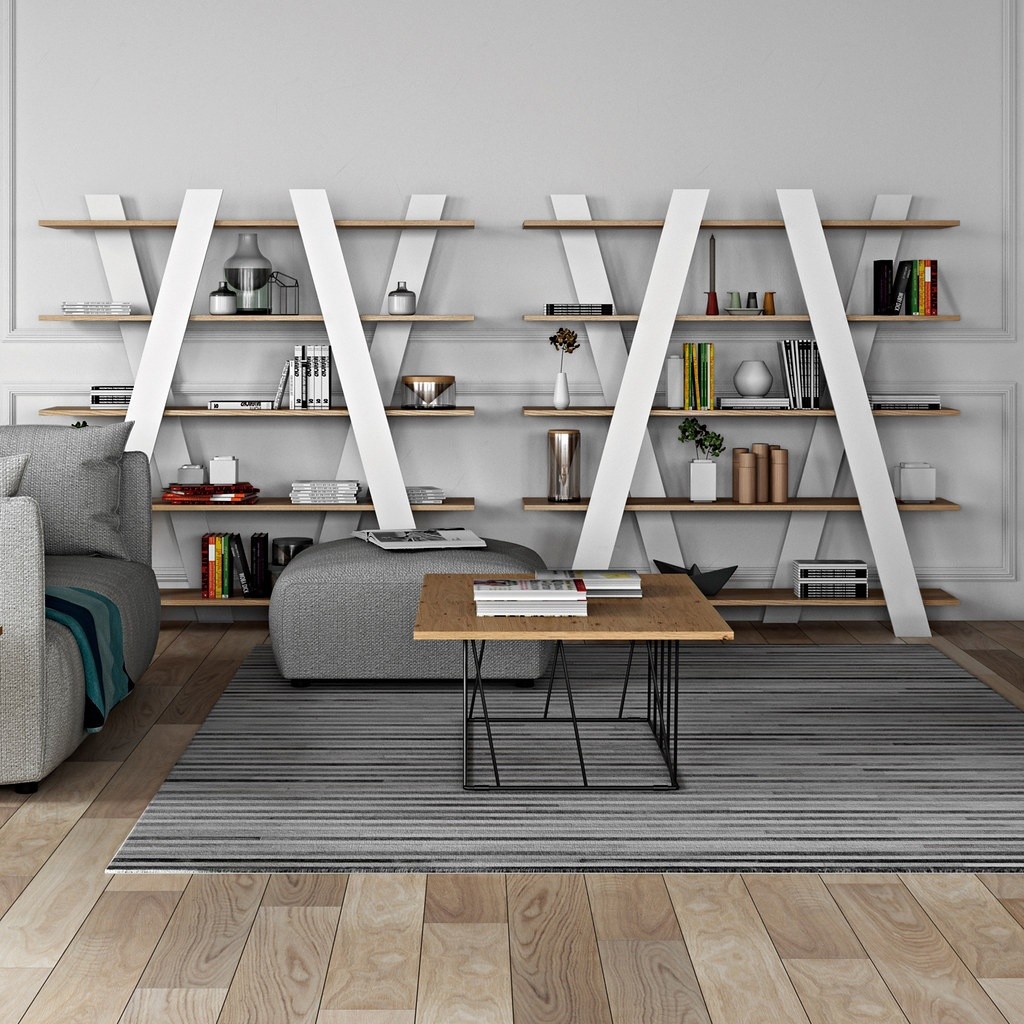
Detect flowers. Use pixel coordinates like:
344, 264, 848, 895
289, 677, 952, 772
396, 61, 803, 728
548, 328, 581, 372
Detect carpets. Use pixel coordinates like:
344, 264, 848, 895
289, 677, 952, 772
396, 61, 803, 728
106, 642, 1023, 871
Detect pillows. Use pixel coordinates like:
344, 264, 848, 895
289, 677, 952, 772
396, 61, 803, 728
0, 454, 30, 497
0, 420, 136, 559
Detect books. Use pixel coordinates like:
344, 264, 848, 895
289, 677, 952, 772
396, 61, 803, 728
534, 569, 642, 598
289, 479, 362, 504
868, 394, 941, 410
62, 302, 131, 316
873, 260, 938, 316
272, 345, 332, 410
543, 304, 614, 316
160, 482, 260, 505
777, 339, 827, 410
406, 486, 446, 504
683, 342, 715, 411
207, 401, 272, 410
201, 532, 268, 599
89, 385, 134, 409
350, 527, 487, 550
472, 579, 588, 617
792, 560, 868, 598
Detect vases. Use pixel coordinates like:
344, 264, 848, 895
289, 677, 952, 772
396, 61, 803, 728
554, 370, 571, 411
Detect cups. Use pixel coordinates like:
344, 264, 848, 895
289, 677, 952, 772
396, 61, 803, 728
732, 442, 787, 506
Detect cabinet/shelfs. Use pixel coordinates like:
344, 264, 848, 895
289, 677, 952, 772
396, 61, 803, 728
523, 190, 962, 637
39, 187, 474, 607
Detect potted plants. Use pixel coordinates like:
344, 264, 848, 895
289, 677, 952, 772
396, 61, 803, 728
678, 417, 726, 503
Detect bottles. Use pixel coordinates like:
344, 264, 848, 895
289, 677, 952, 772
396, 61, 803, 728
224, 233, 272, 314
388, 281, 417, 315
208, 281, 237, 315
733, 361, 772, 398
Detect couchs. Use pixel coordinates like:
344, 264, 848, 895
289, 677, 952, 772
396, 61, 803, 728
0, 451, 161, 795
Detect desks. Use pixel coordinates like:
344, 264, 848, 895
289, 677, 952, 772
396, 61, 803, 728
414, 574, 734, 791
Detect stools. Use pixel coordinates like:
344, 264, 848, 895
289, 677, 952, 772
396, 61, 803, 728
269, 539, 554, 687
